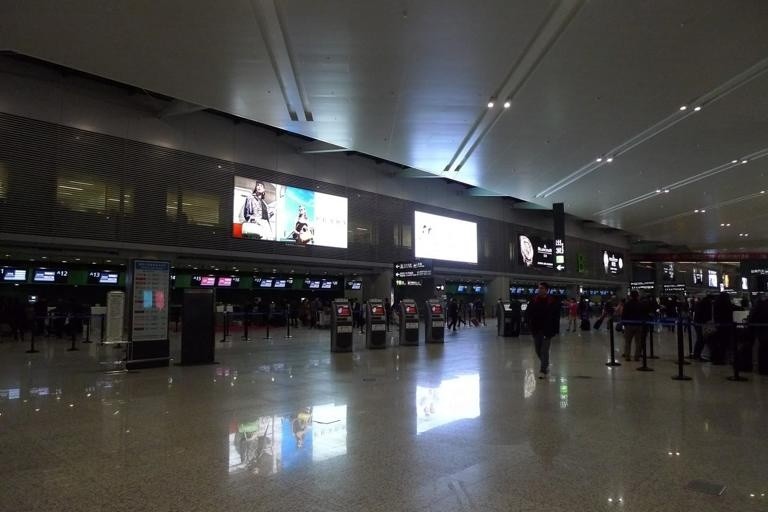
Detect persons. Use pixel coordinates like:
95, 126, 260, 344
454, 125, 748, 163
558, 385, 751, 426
289, 407, 311, 448
510, 296, 521, 337
244, 183, 273, 232
2, 296, 83, 341
562, 290, 768, 374
250, 295, 487, 333
529, 378, 569, 473
240, 425, 270, 480
524, 283, 560, 378
289, 205, 309, 239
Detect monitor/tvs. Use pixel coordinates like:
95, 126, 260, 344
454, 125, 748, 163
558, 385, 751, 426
457, 285, 482, 293
520, 302, 528, 311
199, 275, 363, 291
429, 302, 443, 315
0, 265, 119, 284
510, 287, 614, 295
502, 302, 513, 312
369, 302, 385, 315
403, 302, 419, 314
334, 301, 352, 317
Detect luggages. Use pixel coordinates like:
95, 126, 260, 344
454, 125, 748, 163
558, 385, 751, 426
580, 319, 591, 332
593, 318, 604, 330
471, 319, 479, 327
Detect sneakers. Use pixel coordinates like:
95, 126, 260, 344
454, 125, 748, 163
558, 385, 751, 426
538, 372, 548, 381
